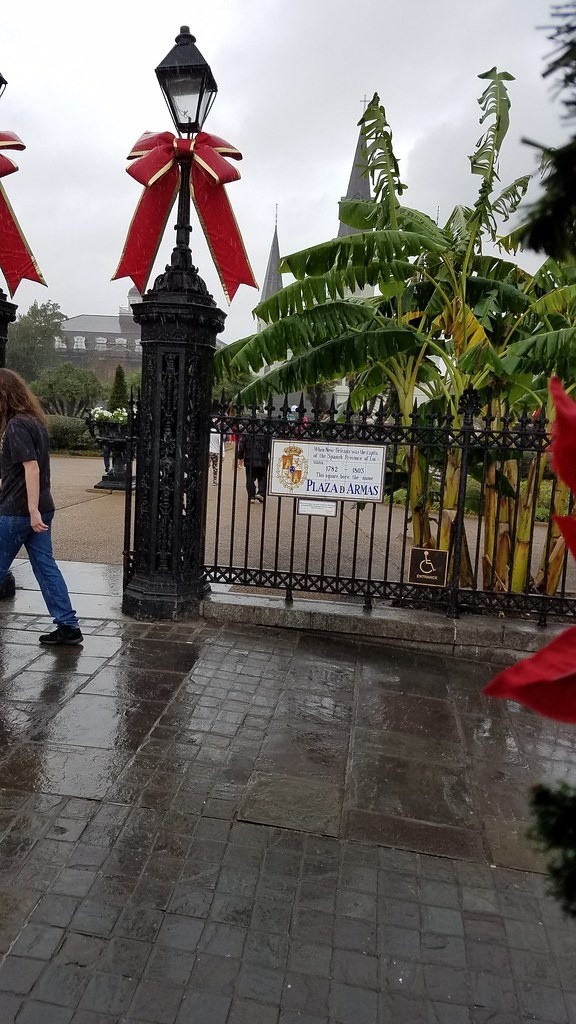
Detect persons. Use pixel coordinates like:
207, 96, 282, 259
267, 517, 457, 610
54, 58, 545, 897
207, 418, 226, 486
0, 369, 84, 645
230, 416, 246, 469
236, 418, 271, 504
295, 411, 309, 437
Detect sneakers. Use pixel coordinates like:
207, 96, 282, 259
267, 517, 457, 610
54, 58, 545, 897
39, 626, 85, 645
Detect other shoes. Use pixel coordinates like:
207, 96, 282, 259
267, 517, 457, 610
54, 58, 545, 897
254, 493, 265, 501
212, 482, 218, 486
249, 499, 256, 504
